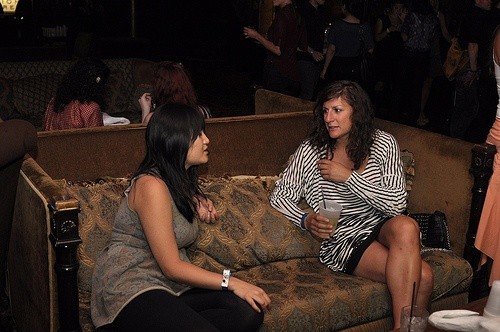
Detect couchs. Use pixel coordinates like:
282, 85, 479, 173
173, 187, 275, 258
1, 58, 160, 128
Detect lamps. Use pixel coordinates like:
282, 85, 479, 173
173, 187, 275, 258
0, 0, 20, 15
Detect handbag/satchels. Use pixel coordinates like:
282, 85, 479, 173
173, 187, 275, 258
409, 210, 451, 252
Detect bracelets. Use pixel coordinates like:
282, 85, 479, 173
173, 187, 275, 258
469, 69, 477, 73
220, 268, 231, 292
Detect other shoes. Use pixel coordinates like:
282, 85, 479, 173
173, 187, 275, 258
417, 118, 429, 126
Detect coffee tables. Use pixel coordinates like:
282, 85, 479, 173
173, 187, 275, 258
390, 293, 491, 332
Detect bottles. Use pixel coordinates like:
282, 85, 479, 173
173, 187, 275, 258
324, 23, 332, 42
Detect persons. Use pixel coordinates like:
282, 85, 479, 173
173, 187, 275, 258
42, 58, 213, 132
243, 0, 500, 145
468, 24, 499, 286
91, 101, 272, 332
269, 79, 436, 332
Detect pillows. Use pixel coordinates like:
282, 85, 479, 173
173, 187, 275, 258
224, 174, 322, 268
54, 172, 235, 305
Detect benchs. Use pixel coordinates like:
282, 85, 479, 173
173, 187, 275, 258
6, 89, 498, 332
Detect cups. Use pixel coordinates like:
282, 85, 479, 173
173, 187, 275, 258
245, 26, 255, 38
483, 280, 500, 320
399, 306, 429, 332
319, 201, 342, 237
